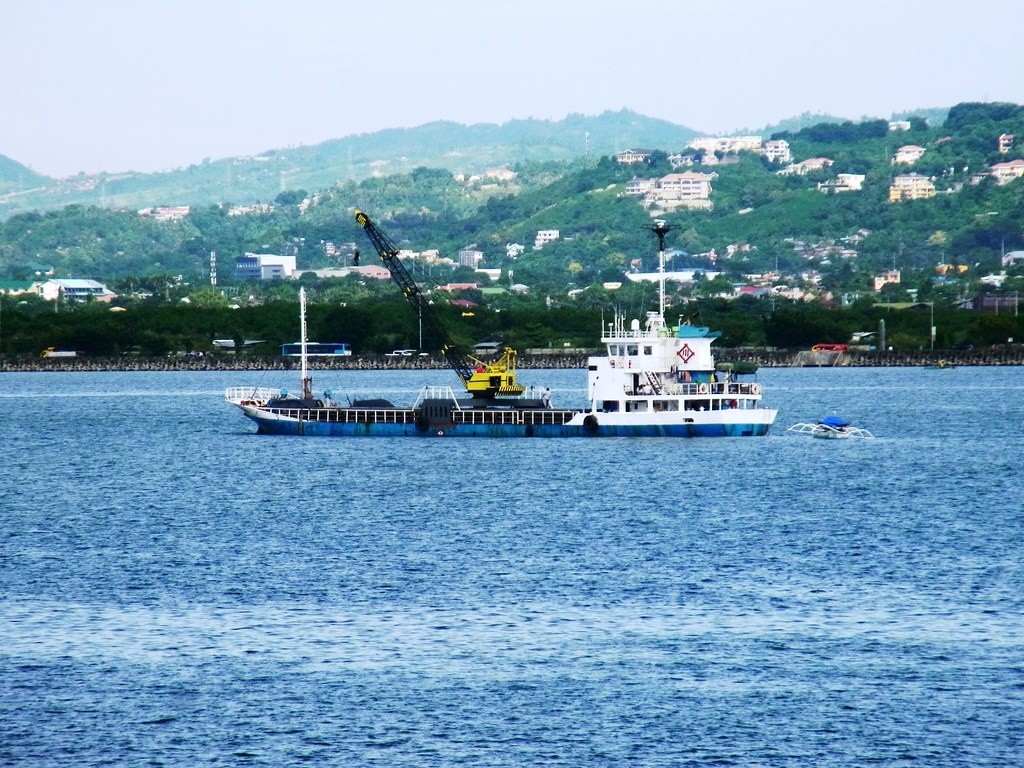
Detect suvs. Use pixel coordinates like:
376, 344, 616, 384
384, 350, 431, 357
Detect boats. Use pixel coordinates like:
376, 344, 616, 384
785, 415, 875, 440
225, 210, 781, 439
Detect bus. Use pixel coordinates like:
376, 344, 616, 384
277, 342, 353, 357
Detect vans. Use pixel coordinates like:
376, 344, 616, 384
472, 342, 504, 358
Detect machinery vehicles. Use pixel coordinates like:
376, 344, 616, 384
352, 210, 528, 396
